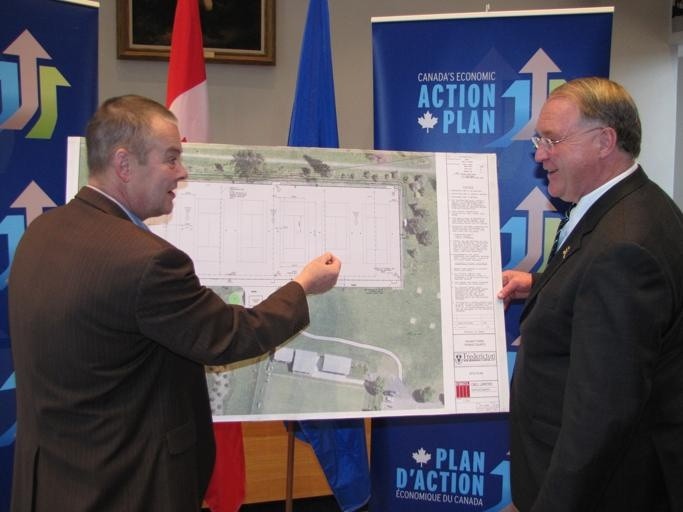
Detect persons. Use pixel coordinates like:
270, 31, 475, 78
7, 94, 341, 512
497, 74, 683, 512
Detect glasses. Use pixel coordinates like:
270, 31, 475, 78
530, 127, 604, 150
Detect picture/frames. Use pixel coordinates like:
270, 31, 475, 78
114, 0, 276, 67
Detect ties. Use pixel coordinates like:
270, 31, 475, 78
548, 203, 577, 266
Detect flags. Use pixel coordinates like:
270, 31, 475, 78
164, 1, 248, 512
280, 1, 372, 510
